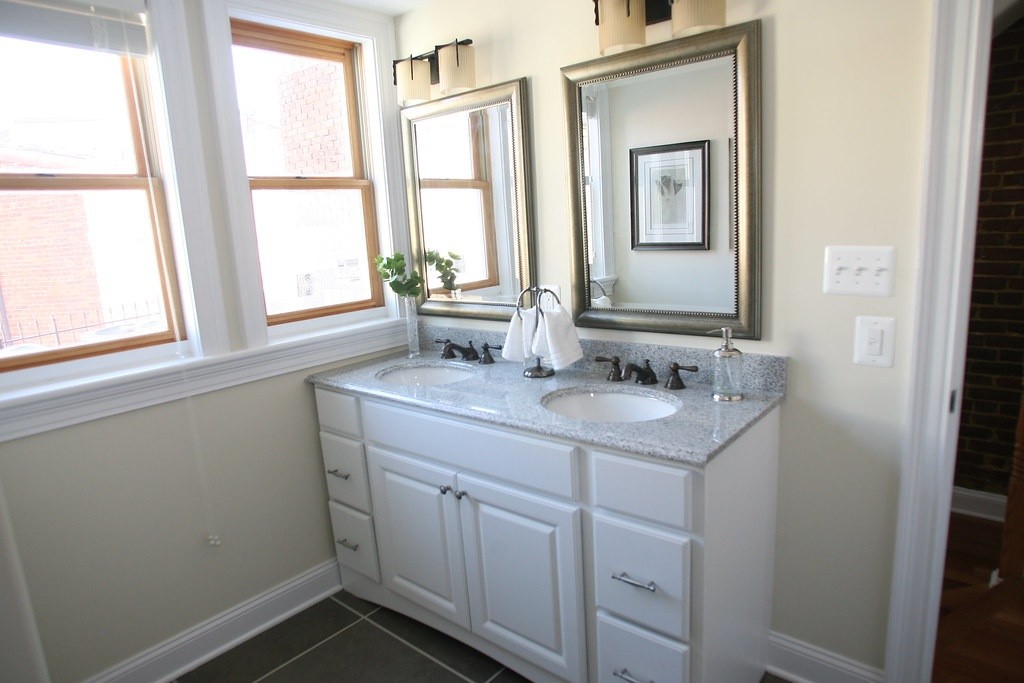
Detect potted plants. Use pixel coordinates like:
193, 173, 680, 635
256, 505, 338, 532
373, 249, 425, 358
425, 249, 461, 301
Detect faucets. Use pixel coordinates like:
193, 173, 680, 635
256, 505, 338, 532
621, 358, 659, 385
440, 341, 479, 361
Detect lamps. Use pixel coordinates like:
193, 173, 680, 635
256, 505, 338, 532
593, 0, 727, 56
392, 39, 476, 106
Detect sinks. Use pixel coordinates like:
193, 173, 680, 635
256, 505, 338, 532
539, 384, 686, 423
374, 357, 478, 386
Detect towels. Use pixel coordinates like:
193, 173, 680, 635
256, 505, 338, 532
590, 295, 612, 308
531, 303, 584, 372
501, 303, 538, 361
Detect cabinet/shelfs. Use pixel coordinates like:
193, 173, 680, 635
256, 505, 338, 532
589, 450, 692, 683
361, 398, 589, 683
314, 386, 380, 583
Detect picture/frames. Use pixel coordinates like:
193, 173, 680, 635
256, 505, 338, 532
629, 139, 710, 251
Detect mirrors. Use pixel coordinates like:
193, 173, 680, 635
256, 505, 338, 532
559, 19, 762, 342
398, 75, 534, 321
